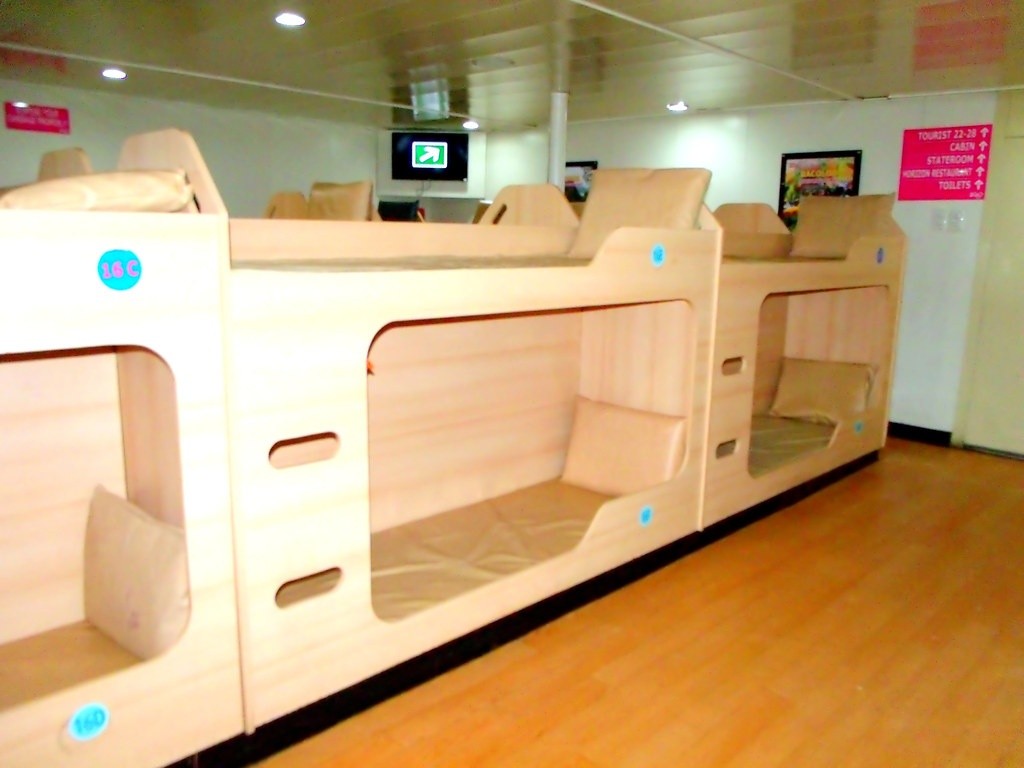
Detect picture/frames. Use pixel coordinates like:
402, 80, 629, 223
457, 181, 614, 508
777, 149, 863, 226
563, 160, 598, 201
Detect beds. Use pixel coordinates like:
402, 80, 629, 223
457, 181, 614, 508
0, 125, 908, 768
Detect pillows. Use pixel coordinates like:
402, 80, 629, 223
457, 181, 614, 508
569, 165, 713, 260
792, 193, 897, 256
302, 181, 373, 223
84, 484, 188, 657
1, 165, 191, 214
769, 356, 875, 427
560, 391, 688, 496
